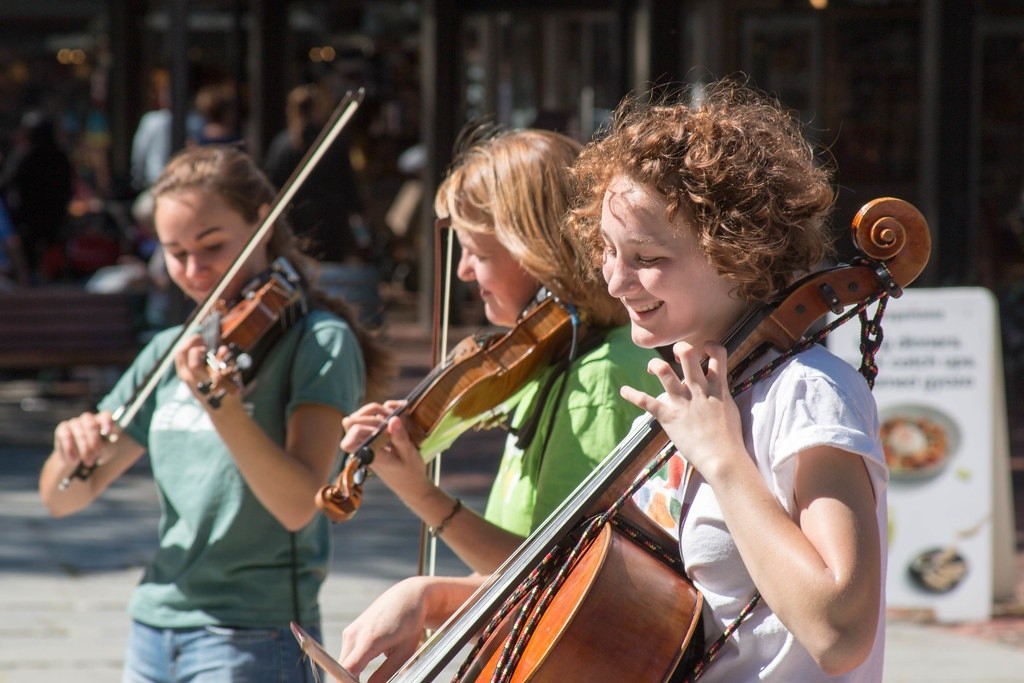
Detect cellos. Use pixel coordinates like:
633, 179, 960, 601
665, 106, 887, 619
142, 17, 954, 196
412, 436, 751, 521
382, 197, 932, 683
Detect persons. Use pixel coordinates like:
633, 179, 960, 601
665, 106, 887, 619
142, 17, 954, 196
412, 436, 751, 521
337, 69, 889, 683
339, 113, 664, 577
38, 143, 403, 683
0, 43, 421, 339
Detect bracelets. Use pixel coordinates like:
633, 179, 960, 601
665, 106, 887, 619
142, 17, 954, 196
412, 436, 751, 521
429, 497, 462, 538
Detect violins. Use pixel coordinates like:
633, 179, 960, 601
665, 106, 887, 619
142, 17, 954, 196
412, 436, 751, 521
314, 295, 590, 527
194, 266, 289, 408
